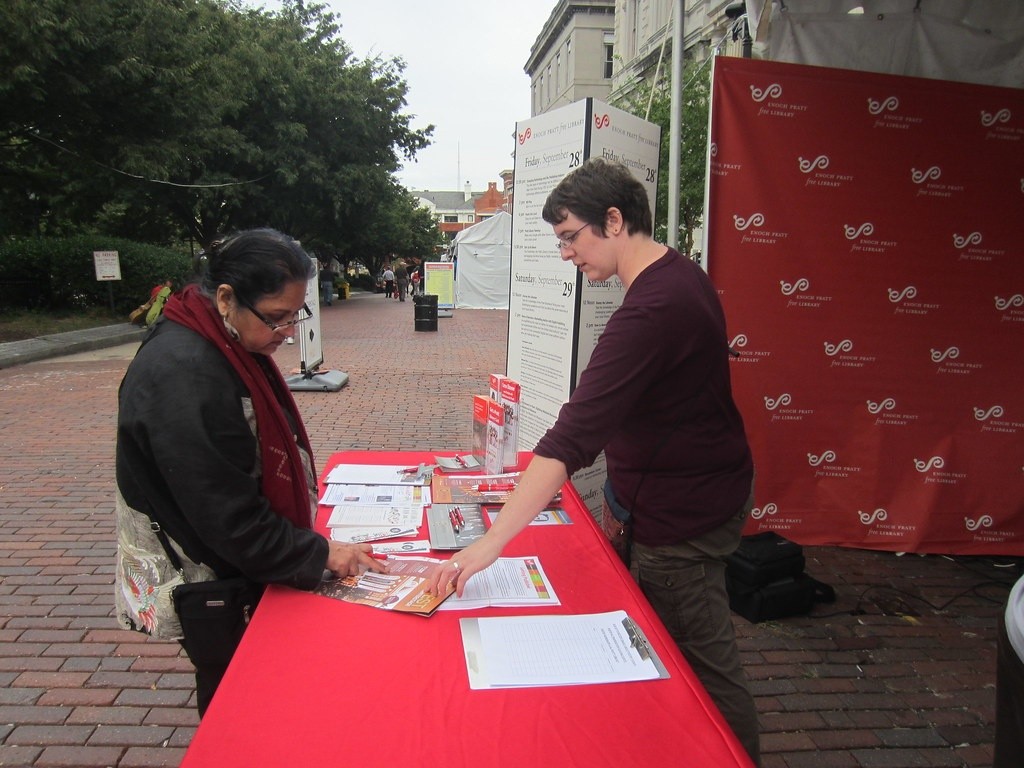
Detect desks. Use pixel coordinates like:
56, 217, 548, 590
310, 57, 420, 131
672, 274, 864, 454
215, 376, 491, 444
179, 450, 757, 768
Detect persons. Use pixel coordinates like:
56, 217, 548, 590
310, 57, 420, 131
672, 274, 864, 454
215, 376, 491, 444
114, 228, 390, 723
424, 157, 761, 768
321, 265, 336, 306
383, 261, 424, 301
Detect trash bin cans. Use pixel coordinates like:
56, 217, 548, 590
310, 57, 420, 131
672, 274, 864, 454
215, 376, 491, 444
412, 295, 439, 332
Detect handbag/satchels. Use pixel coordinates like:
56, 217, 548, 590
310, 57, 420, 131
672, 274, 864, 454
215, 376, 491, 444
394, 289, 398, 299
602, 479, 633, 571
172, 578, 251, 673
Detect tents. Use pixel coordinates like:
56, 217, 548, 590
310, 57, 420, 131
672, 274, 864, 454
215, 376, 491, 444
445, 210, 513, 311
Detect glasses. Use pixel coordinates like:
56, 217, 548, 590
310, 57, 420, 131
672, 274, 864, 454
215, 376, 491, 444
237, 294, 313, 337
555, 220, 593, 249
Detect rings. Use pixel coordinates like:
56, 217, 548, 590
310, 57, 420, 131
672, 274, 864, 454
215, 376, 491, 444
453, 562, 461, 574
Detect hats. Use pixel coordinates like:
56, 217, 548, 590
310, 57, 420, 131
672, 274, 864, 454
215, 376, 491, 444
399, 262, 406, 265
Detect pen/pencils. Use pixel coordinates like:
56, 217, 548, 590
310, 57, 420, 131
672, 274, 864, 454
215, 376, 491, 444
368, 551, 387, 559
469, 487, 516, 491
449, 508, 460, 532
455, 454, 468, 468
454, 507, 466, 527
470, 484, 516, 489
397, 467, 418, 473
